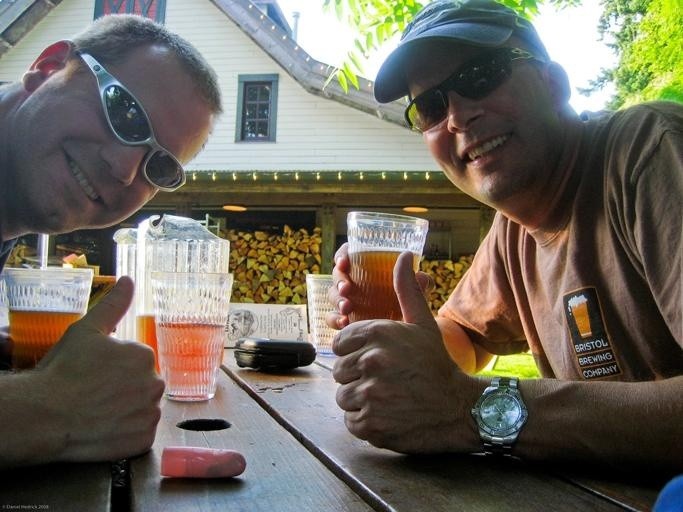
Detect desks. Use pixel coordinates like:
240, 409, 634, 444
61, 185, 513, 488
0, 350, 663, 511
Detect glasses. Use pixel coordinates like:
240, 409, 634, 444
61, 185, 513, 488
79, 52, 187, 194
404, 46, 545, 134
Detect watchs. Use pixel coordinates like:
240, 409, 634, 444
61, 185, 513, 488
470, 373, 528, 471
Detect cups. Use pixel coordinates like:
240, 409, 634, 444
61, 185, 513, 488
306, 273, 342, 359
346, 211, 430, 324
4, 268, 94, 370
150, 270, 234, 402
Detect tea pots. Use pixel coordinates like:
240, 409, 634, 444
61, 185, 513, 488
136, 214, 232, 375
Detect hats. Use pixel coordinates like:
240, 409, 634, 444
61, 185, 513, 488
372, 0, 551, 105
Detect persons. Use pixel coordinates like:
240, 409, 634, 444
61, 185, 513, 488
320, 1, 683, 485
1, 8, 223, 474
